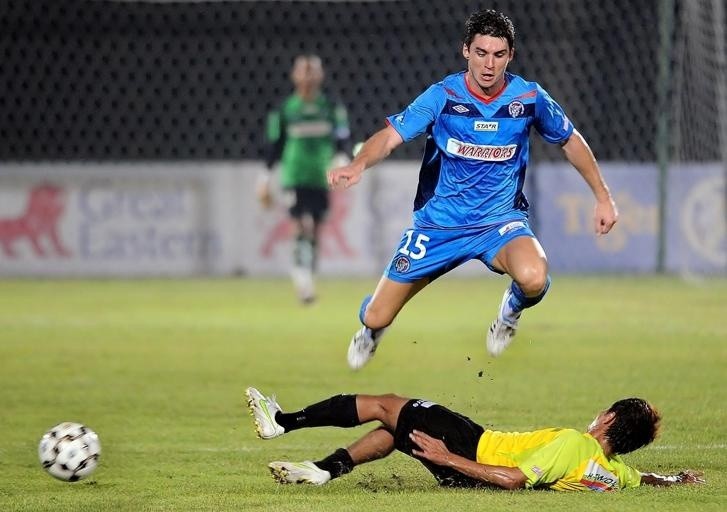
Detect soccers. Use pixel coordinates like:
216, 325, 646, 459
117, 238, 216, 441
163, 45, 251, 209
37, 421, 100, 482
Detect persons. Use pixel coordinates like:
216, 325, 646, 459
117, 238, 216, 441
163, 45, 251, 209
259, 53, 352, 303
326, 11, 619, 372
243, 388, 706, 494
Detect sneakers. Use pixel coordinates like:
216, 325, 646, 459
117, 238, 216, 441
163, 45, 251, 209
267, 461, 331, 485
486, 288, 522, 354
347, 323, 384, 369
247, 386, 285, 440
